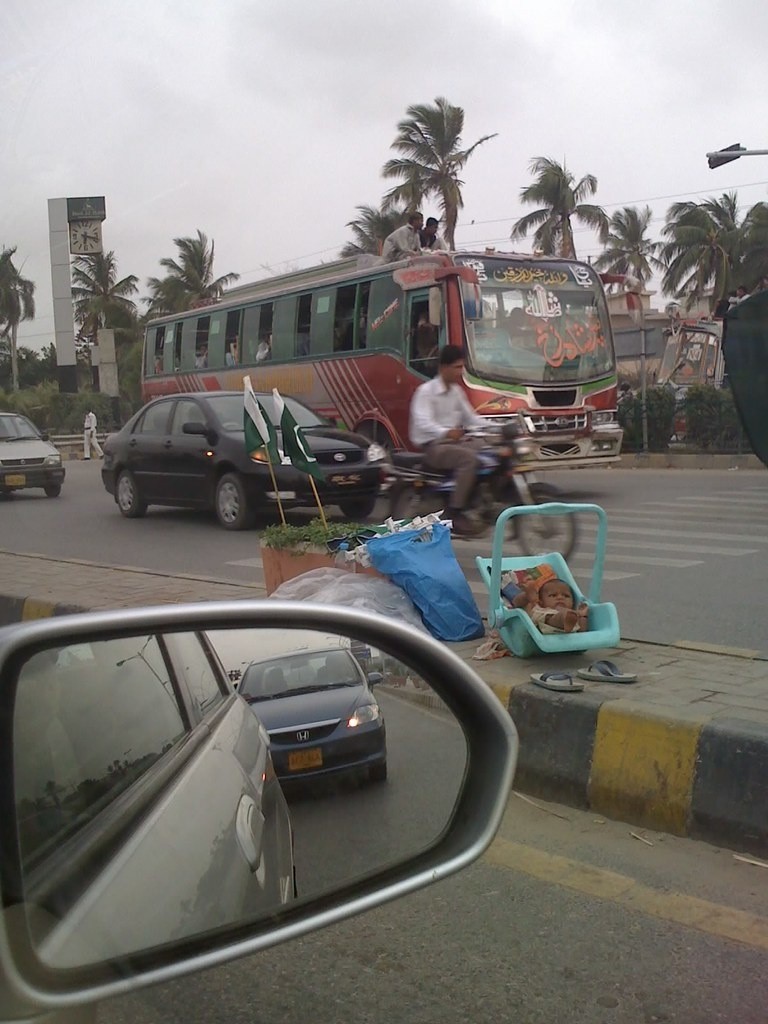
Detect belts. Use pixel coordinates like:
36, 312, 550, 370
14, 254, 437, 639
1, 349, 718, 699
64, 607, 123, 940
85, 427, 97, 429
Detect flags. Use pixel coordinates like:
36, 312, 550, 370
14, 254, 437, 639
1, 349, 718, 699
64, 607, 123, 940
242, 375, 327, 485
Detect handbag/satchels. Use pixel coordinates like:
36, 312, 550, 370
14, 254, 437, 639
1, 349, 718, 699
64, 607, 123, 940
367, 522, 485, 641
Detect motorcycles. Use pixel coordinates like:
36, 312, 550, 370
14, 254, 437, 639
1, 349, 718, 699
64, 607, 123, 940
366, 416, 577, 562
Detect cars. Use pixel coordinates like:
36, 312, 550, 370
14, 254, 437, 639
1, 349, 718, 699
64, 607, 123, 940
101, 390, 384, 531
0, 599, 520, 1024
0, 412, 66, 498
235, 645, 387, 782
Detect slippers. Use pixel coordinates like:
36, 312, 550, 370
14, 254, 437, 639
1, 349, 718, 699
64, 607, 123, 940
578, 660, 637, 681
529, 672, 584, 690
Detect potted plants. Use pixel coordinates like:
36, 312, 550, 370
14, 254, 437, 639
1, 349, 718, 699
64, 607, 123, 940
259, 509, 403, 598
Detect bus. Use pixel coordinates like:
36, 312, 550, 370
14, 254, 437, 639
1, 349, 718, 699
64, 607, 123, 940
140, 246, 625, 499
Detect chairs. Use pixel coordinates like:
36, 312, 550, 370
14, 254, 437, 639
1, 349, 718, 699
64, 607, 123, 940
263, 666, 288, 693
317, 666, 329, 684
296, 333, 310, 356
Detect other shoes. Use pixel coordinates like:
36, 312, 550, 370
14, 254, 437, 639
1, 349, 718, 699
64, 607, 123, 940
447, 513, 488, 535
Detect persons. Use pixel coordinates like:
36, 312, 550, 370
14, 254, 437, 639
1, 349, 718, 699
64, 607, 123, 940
382, 212, 423, 263
727, 285, 751, 312
195, 342, 208, 369
688, 348, 700, 361
359, 305, 367, 323
617, 380, 635, 408
414, 310, 439, 371
504, 306, 527, 338
408, 344, 524, 534
80, 406, 105, 460
420, 217, 439, 249
155, 356, 163, 374
258, 332, 272, 361
341, 319, 354, 350
652, 367, 657, 389
531, 579, 590, 633
